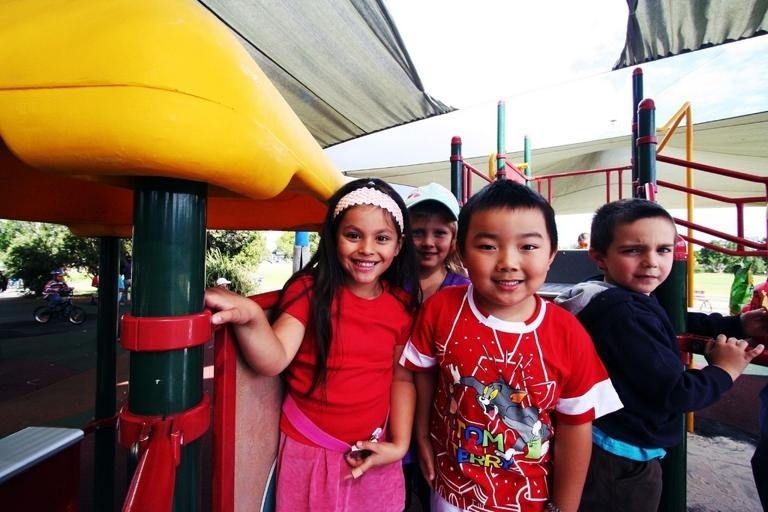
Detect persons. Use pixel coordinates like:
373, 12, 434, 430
41, 267, 75, 312
553, 198, 767, 511
204, 178, 417, 511
397, 179, 625, 512
213, 276, 233, 290
399, 183, 472, 511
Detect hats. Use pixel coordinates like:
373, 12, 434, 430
405, 182, 462, 222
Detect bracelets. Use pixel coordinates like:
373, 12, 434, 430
545, 499, 560, 512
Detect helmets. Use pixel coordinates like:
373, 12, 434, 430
50, 266, 63, 278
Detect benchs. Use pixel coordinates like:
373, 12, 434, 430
0, 426, 85, 512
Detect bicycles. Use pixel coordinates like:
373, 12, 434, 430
33, 288, 87, 325
